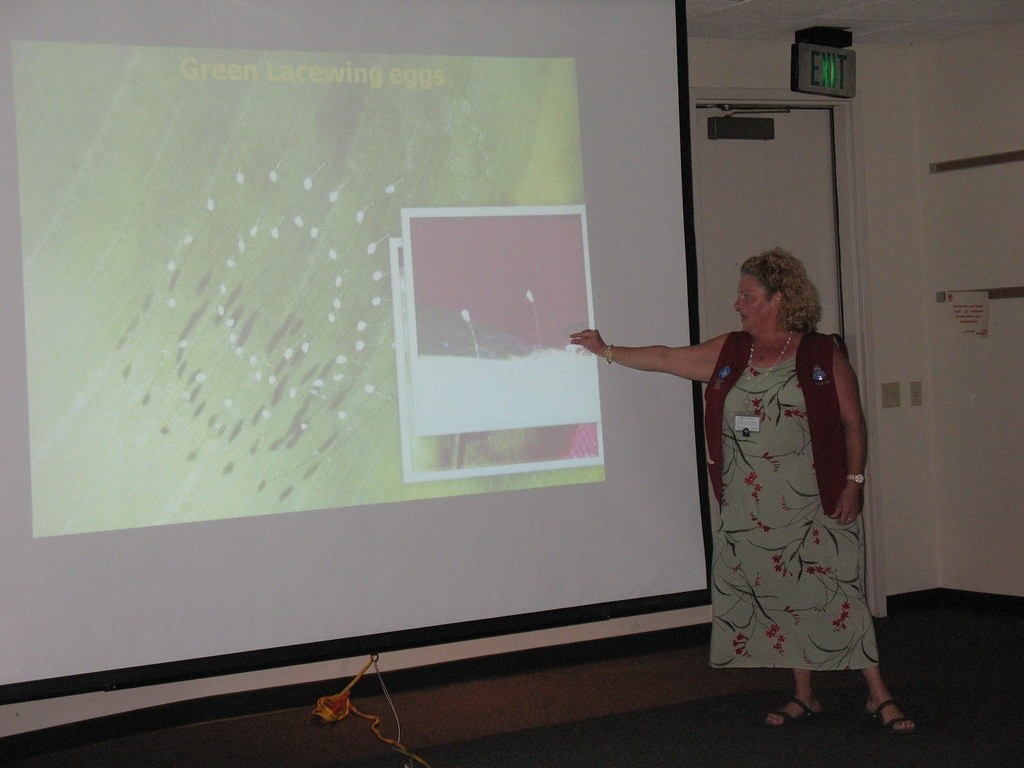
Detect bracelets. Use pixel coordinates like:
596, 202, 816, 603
604, 344, 614, 364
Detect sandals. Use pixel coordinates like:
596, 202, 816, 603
864, 698, 916, 736
765, 691, 824, 727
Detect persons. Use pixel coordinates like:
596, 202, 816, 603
569, 246, 916, 734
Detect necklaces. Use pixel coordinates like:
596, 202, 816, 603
743, 331, 792, 405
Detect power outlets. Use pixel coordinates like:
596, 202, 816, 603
910, 382, 922, 406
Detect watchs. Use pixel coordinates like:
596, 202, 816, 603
846, 474, 864, 483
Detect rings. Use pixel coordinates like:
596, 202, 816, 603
848, 518, 853, 521
